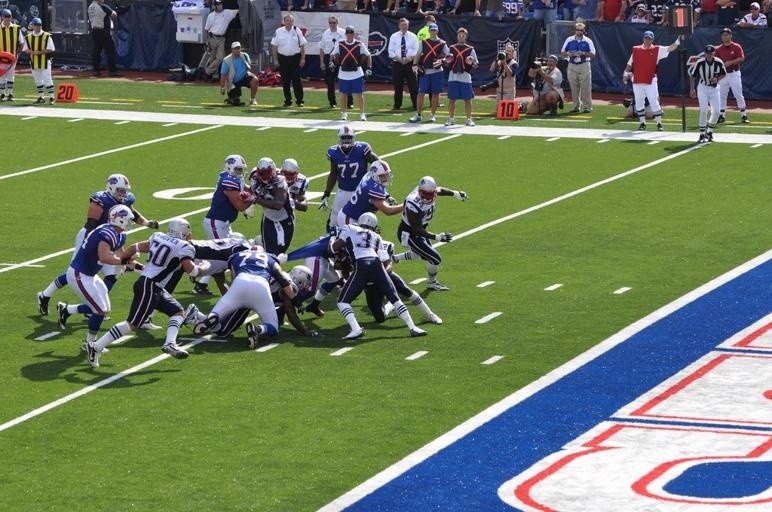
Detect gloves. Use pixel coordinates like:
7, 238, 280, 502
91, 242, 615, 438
148, 221, 158, 229
298, 306, 306, 314
454, 190, 470, 202
435, 232, 453, 242
318, 195, 329, 211
387, 197, 398, 205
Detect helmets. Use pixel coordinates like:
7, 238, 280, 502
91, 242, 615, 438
225, 155, 300, 183
359, 212, 378, 230
214, 0, 222, 5
108, 204, 136, 233
105, 173, 132, 204
339, 128, 356, 152
370, 159, 393, 188
32, 17, 41, 25
418, 176, 436, 209
288, 265, 314, 298
3, 9, 12, 18
167, 217, 192, 244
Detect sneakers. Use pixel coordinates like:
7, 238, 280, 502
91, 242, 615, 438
50, 97, 55, 104
410, 327, 427, 335
584, 109, 591, 113
284, 102, 292, 106
637, 116, 750, 142
33, 96, 45, 104
341, 112, 347, 120
192, 281, 213, 295
550, 105, 557, 115
299, 103, 304, 107
250, 98, 257, 105
572, 109, 579, 112
423, 312, 442, 323
409, 114, 475, 126
306, 304, 325, 316
427, 280, 449, 290
8, 94, 14, 101
37, 292, 217, 366
360, 113, 366, 121
343, 327, 365, 339
0, 94, 7, 101
246, 322, 262, 349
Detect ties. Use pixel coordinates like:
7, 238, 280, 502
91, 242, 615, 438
401, 33, 406, 57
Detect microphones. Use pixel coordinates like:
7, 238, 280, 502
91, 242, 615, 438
581, 32, 585, 36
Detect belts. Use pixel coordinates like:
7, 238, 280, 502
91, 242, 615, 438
726, 69, 739, 73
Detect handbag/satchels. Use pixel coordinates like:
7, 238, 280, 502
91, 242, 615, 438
93, 28, 112, 44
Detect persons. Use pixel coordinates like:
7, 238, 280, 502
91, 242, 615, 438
561, 23, 596, 113
360, 239, 444, 326
388, 16, 419, 110
37, 173, 159, 322
484, 0, 772, 32
409, 30, 449, 123
330, 26, 372, 122
176, 265, 324, 339
444, 26, 480, 126
56, 206, 144, 355
192, 153, 252, 296
690, 44, 726, 144
81, 215, 207, 370
137, 232, 269, 329
272, 14, 307, 107
220, 41, 259, 107
276, 157, 310, 218
23, 17, 55, 106
199, 0, 240, 80
528, 55, 565, 117
336, 160, 406, 227
416, 14, 444, 109
390, 176, 466, 291
290, 0, 483, 15
713, 27, 748, 124
0, 9, 24, 102
195, 243, 293, 350
316, 125, 379, 229
240, 158, 295, 255
622, 31, 685, 132
88, 0, 125, 77
286, 225, 380, 317
319, 16, 355, 109
490, 45, 529, 114
328, 213, 428, 340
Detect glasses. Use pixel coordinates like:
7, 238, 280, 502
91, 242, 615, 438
575, 28, 583, 32
346, 31, 352, 34
329, 22, 337, 25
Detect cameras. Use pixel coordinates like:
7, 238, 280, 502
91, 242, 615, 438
497, 52, 507, 60
479, 79, 499, 92
531, 57, 548, 69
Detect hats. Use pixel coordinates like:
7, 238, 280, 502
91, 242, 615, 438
429, 23, 438, 31
704, 45, 715, 52
457, 28, 468, 35
635, 5, 645, 12
644, 31, 654, 39
720, 28, 732, 34
231, 42, 241, 47
750, 2, 760, 9
346, 27, 353, 32
